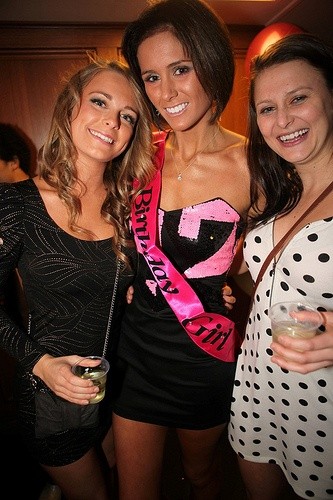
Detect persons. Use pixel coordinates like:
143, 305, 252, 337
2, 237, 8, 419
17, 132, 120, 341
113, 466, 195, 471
1, 123, 33, 185
0, 61, 235, 500
126, 33, 333, 500
114, 2, 266, 500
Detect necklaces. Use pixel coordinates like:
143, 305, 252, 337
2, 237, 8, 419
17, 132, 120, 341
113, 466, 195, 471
168, 126, 222, 181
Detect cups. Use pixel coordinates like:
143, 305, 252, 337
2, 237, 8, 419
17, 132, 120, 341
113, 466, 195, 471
268, 301, 324, 366
72, 357, 109, 405
